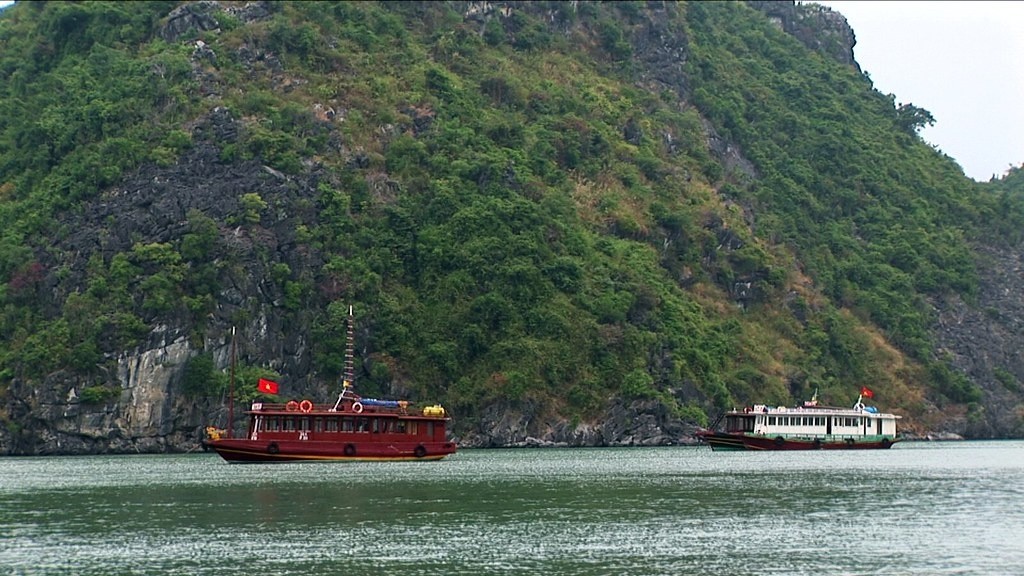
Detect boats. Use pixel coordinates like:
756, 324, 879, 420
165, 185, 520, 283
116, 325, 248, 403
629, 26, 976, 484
201, 304, 458, 465
694, 387, 904, 451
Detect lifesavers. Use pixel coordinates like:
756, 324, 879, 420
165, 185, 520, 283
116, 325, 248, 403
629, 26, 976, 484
814, 440, 820, 449
847, 438, 855, 447
267, 442, 280, 454
285, 400, 299, 412
414, 446, 427, 457
352, 402, 363, 413
882, 438, 889, 445
299, 400, 313, 412
344, 444, 356, 456
774, 436, 785, 446
